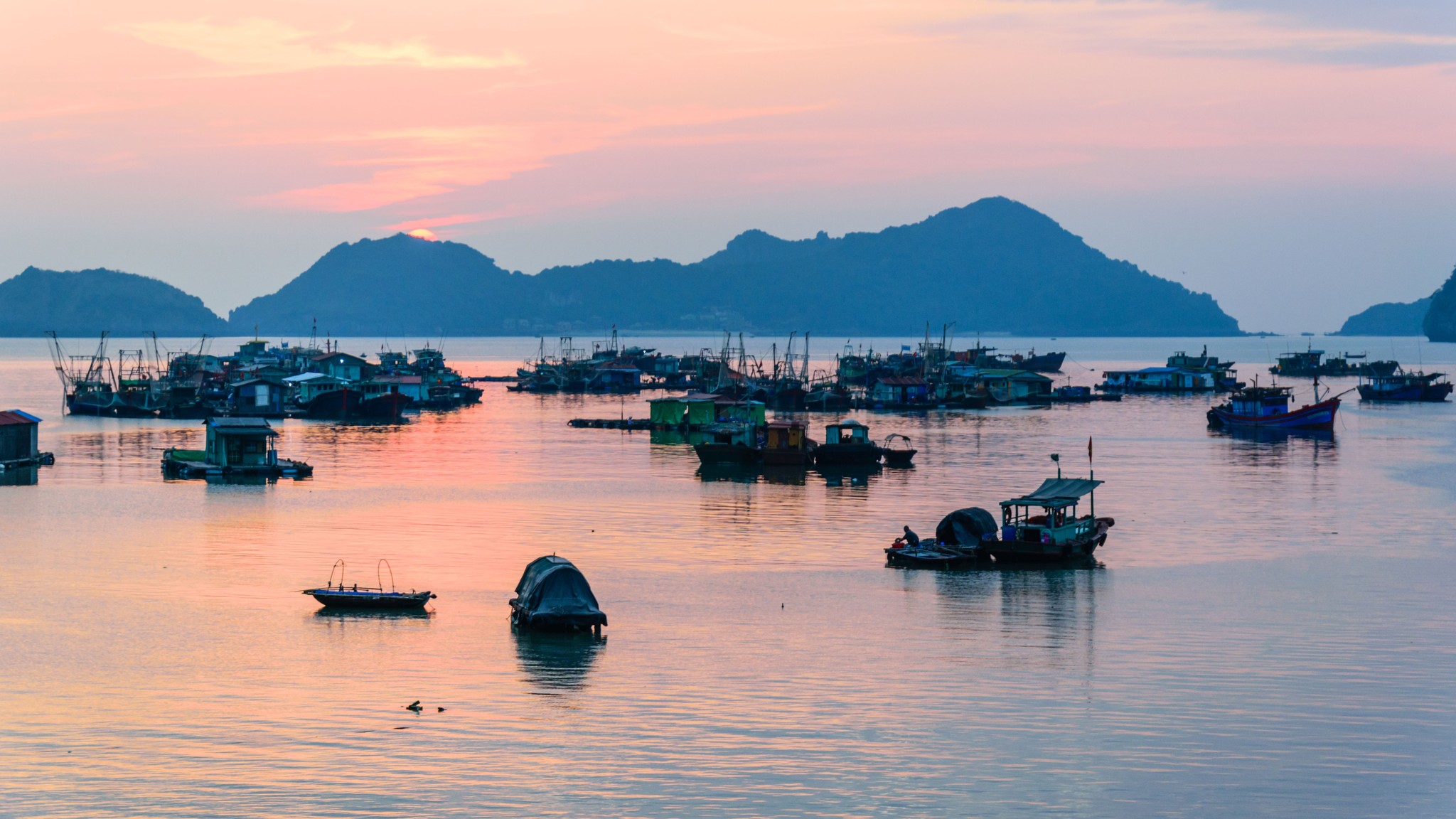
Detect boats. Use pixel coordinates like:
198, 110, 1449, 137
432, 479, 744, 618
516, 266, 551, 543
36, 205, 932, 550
883, 476, 1115, 572
756, 419, 818, 467
1267, 335, 1400, 376
882, 433, 918, 464
813, 419, 883, 466
158, 415, 315, 485
42, 315, 485, 425
1344, 351, 1367, 358
509, 554, 608, 634
301, 558, 438, 610
505, 319, 1247, 431
1354, 371, 1455, 403
1206, 372, 1357, 433
693, 387, 768, 467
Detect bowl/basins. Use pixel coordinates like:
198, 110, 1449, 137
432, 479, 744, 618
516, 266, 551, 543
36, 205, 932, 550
891, 543, 905, 548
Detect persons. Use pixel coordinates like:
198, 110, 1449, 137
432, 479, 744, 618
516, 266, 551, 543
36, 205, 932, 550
895, 526, 919, 548
842, 435, 852, 443
866, 387, 880, 399
1218, 402, 1233, 413
910, 398, 916, 403
651, 376, 662, 383
628, 416, 634, 425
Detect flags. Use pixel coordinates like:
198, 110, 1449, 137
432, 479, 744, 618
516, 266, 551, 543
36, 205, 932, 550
1088, 435, 1093, 463
381, 344, 384, 350
597, 344, 600, 349
441, 358, 447, 363
963, 367, 965, 370
759, 361, 762, 368
314, 317, 316, 322
612, 324, 616, 329
913, 352, 918, 357
427, 342, 429, 347
848, 338, 862, 348
437, 378, 443, 385
